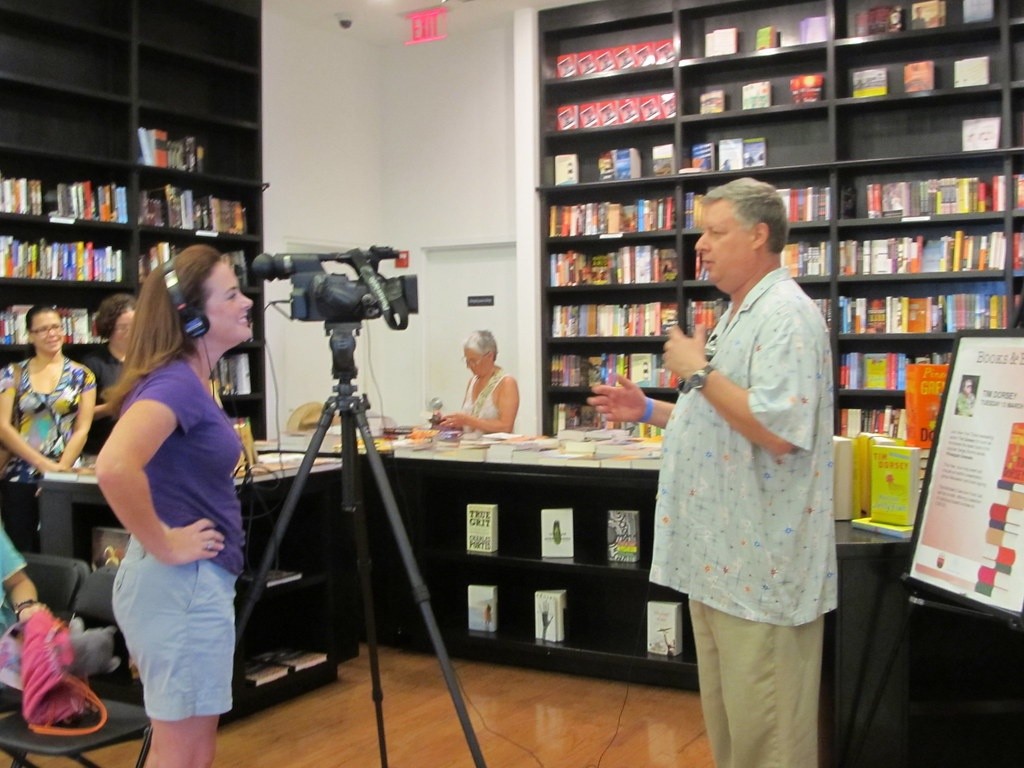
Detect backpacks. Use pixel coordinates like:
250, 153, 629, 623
22, 610, 106, 736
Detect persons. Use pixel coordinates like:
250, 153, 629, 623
95, 245, 256, 768
0, 527, 122, 706
955, 379, 975, 417
83, 294, 141, 458
439, 331, 520, 434
0, 305, 98, 554
587, 178, 837, 768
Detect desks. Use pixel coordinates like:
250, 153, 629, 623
254, 431, 699, 692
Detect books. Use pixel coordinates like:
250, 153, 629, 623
333, 403, 667, 465
0, 180, 253, 473
137, 127, 205, 173
552, 1, 1023, 539
466, 504, 684, 656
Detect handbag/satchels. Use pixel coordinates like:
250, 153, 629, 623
0, 363, 21, 479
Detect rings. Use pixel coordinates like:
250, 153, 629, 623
207, 540, 213, 550
450, 422, 455, 427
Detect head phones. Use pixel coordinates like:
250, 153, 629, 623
163, 257, 209, 338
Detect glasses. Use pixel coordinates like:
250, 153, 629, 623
28, 324, 63, 335
114, 327, 131, 333
461, 351, 491, 367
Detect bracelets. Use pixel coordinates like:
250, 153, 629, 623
13, 599, 39, 620
639, 398, 654, 423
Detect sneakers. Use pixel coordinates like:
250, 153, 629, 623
71, 617, 83, 635
103, 656, 120, 672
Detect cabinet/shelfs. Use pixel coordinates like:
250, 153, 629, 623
37, 456, 359, 730
0, 0, 267, 455
535, 0, 1024, 440
817, 520, 1024, 768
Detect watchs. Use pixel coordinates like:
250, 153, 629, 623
682, 364, 714, 394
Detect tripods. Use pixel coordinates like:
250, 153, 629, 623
135, 321, 487, 768
